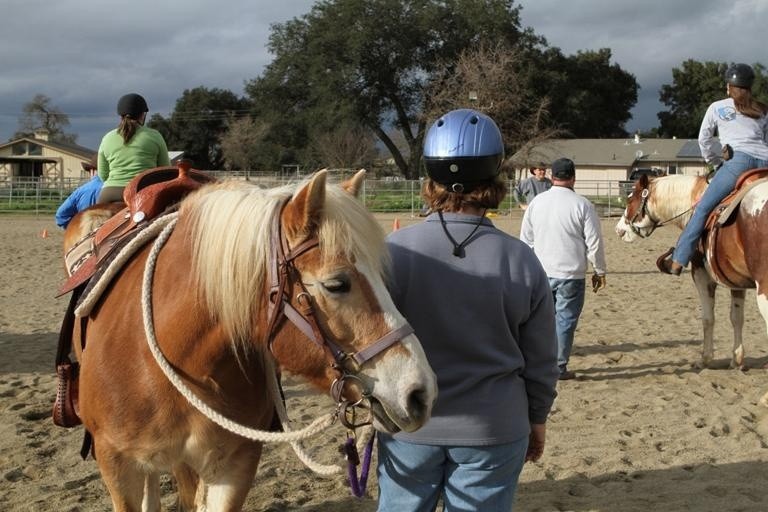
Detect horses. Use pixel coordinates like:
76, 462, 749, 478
63, 201, 125, 277
61, 167, 440, 512
615, 169, 768, 370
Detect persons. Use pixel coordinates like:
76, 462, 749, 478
96, 94, 173, 202
359, 109, 557, 510
660, 63, 767, 276
513, 163, 550, 211
519, 159, 605, 378
56, 158, 107, 230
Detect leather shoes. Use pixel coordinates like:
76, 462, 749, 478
662, 261, 685, 276
556, 368, 576, 380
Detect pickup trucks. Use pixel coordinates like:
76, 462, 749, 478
617, 166, 664, 199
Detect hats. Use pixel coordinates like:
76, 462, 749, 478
551, 157, 576, 180
530, 161, 547, 170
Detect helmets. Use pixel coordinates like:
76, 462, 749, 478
116, 93, 150, 117
723, 64, 757, 89
420, 108, 505, 191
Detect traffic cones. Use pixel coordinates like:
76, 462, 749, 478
41, 230, 48, 239
391, 218, 400, 231
